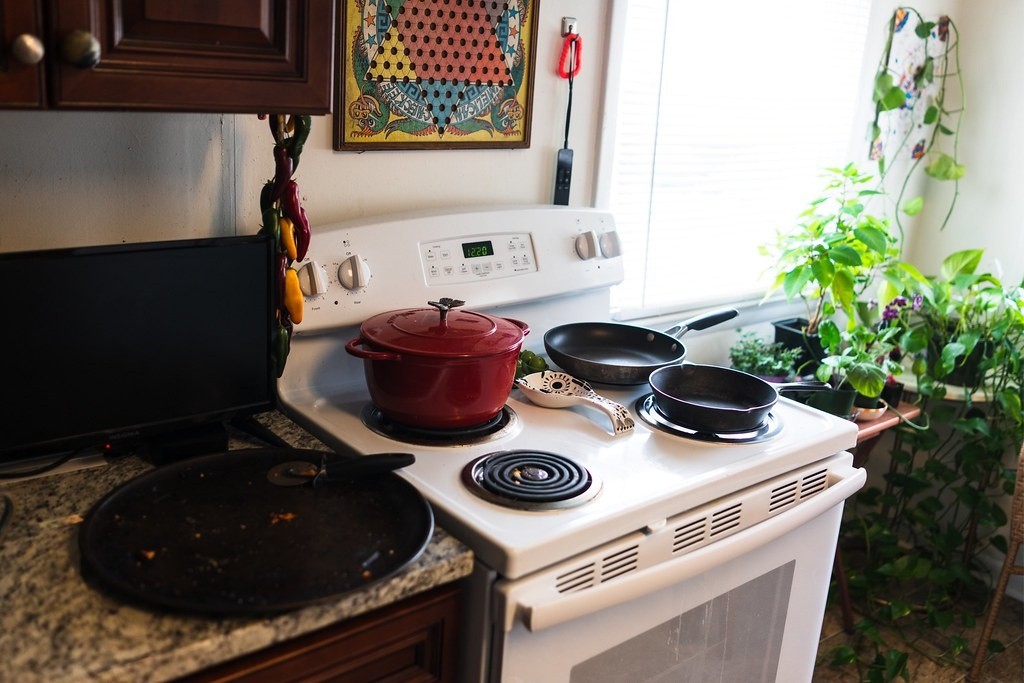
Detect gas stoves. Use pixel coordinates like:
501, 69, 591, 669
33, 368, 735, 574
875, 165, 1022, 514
276, 202, 858, 583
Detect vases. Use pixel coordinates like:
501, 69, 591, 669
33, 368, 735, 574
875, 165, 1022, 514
879, 383, 903, 409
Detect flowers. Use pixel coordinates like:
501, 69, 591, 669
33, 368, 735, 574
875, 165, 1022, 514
856, 294, 923, 384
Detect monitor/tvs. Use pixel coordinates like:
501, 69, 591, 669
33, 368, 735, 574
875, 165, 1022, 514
0, 232, 294, 463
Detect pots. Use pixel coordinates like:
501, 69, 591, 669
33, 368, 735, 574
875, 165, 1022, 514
543, 308, 738, 386
343, 297, 530, 426
648, 362, 832, 431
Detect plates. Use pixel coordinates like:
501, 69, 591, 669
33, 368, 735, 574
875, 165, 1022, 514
515, 369, 636, 435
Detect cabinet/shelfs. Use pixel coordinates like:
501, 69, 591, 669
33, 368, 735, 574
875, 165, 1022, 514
178, 582, 466, 683
0, 0, 334, 115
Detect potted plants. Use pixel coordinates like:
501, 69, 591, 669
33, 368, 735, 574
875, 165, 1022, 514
810, 246, 1023, 683
726, 330, 803, 382
776, 320, 904, 417
759, 161, 934, 376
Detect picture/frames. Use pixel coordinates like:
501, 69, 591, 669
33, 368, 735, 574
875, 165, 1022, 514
333, 0, 540, 151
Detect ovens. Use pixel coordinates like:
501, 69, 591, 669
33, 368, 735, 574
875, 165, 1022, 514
460, 447, 867, 683
